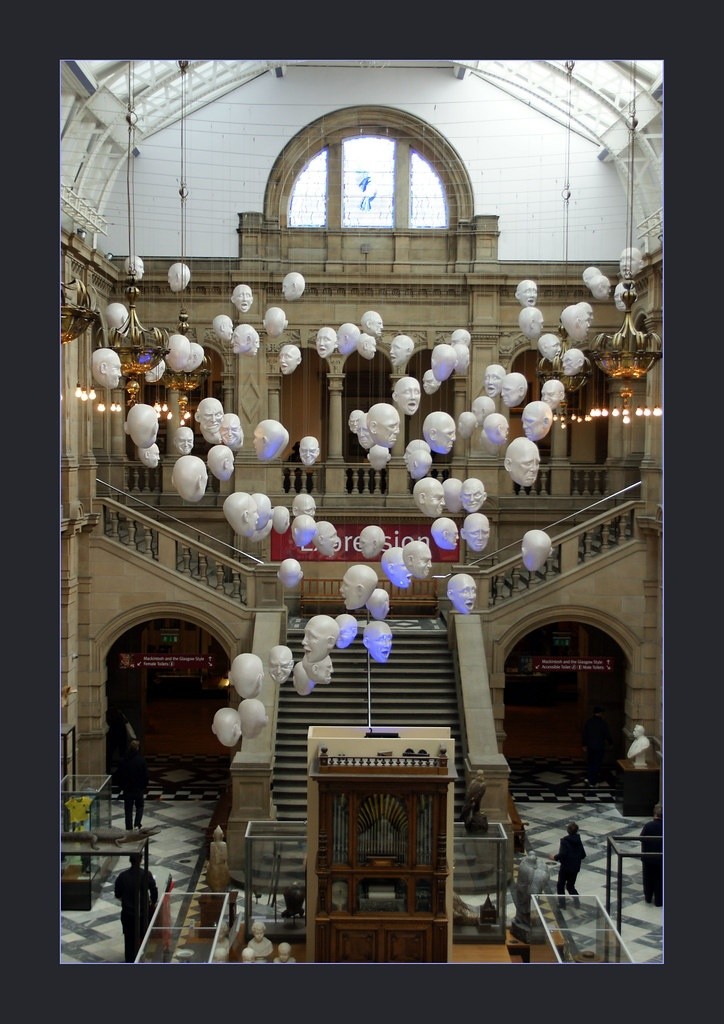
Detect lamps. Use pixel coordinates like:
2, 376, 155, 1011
551, 61, 663, 429
60, 59, 209, 423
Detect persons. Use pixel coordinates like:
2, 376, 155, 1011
273, 942, 296, 963
548, 821, 587, 908
640, 805, 663, 908
116, 740, 148, 830
91, 247, 642, 618
627, 724, 650, 758
269, 613, 393, 695
212, 652, 269, 748
248, 921, 274, 958
214, 922, 229, 964
115, 852, 158, 962
242, 947, 255, 963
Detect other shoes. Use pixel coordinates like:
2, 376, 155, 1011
573, 896, 581, 908
557, 903, 566, 910
